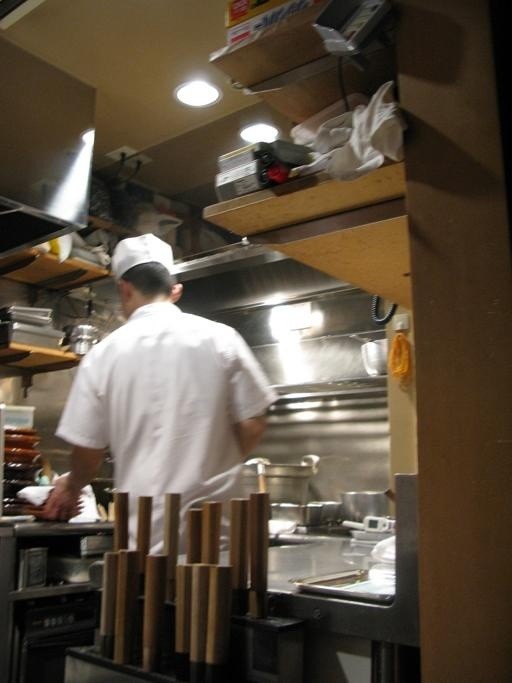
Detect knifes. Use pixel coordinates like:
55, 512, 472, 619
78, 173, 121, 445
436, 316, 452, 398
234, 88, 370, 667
97, 491, 271, 683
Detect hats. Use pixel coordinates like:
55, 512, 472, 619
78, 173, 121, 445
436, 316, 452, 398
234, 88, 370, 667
112, 233, 174, 279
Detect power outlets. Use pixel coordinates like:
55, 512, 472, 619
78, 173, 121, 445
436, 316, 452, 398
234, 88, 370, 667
109, 146, 135, 162
125, 154, 150, 170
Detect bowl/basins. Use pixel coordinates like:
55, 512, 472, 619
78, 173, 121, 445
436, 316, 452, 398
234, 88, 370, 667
339, 490, 388, 524
362, 338, 389, 377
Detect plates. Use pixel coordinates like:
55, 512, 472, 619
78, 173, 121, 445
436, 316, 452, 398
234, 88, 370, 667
2, 429, 84, 524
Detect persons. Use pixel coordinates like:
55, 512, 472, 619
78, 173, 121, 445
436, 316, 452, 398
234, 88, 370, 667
43, 228, 281, 611
